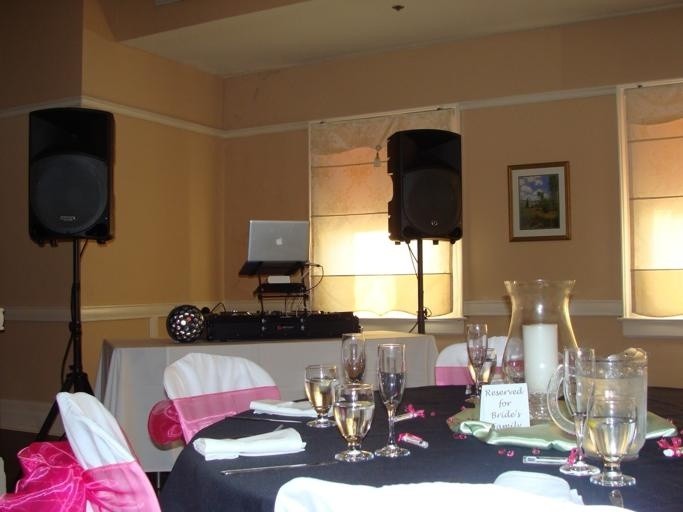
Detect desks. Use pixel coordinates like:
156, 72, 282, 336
103, 330, 435, 493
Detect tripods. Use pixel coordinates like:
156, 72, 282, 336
387, 129, 463, 245
38, 240, 95, 441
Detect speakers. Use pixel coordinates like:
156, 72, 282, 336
28, 107, 115, 248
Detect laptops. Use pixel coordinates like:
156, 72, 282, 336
238, 220, 310, 277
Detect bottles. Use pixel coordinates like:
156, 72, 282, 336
501, 278, 581, 425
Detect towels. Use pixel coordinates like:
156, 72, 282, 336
249, 399, 318, 418
273, 475, 634, 511
192, 427, 306, 461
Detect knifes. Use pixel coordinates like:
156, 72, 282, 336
224, 414, 303, 423
219, 460, 338, 476
609, 489, 624, 508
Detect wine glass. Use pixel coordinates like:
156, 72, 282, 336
587, 396, 638, 487
375, 343, 410, 459
333, 382, 375, 462
465, 323, 496, 404
341, 333, 365, 381
304, 365, 337, 429
559, 348, 599, 475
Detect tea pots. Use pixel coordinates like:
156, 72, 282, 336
546, 345, 647, 462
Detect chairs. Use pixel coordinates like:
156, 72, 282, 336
433, 335, 527, 386
56, 392, 163, 512
163, 352, 283, 444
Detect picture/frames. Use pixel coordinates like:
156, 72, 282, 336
507, 160, 571, 241
